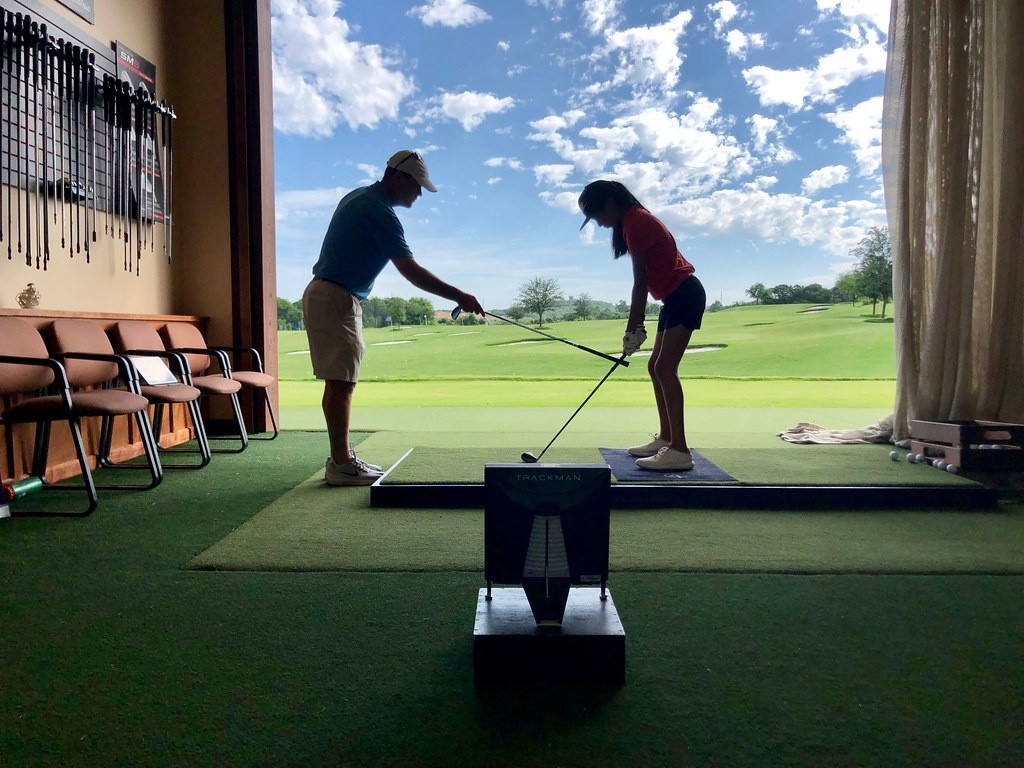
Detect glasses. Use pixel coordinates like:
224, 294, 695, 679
391, 150, 425, 174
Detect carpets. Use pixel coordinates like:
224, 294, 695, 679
598, 447, 739, 481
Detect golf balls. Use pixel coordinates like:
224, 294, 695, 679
889, 451, 899, 460
970, 443, 1002, 449
527, 452, 533, 456
906, 453, 915, 463
932, 459, 957, 473
916, 453, 924, 462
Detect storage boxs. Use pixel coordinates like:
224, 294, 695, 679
911, 419, 1024, 505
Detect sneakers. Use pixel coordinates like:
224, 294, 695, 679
325, 441, 383, 480
327, 459, 385, 486
635, 446, 695, 470
628, 433, 671, 456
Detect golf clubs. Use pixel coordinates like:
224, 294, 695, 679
520, 329, 649, 463
451, 304, 631, 367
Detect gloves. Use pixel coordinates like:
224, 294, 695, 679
623, 331, 640, 356
635, 322, 647, 345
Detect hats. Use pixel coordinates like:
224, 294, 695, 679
577, 180, 610, 231
386, 149, 438, 193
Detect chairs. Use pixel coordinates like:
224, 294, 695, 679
0, 316, 279, 517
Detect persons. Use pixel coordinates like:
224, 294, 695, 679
302, 150, 486, 484
578, 179, 706, 469
